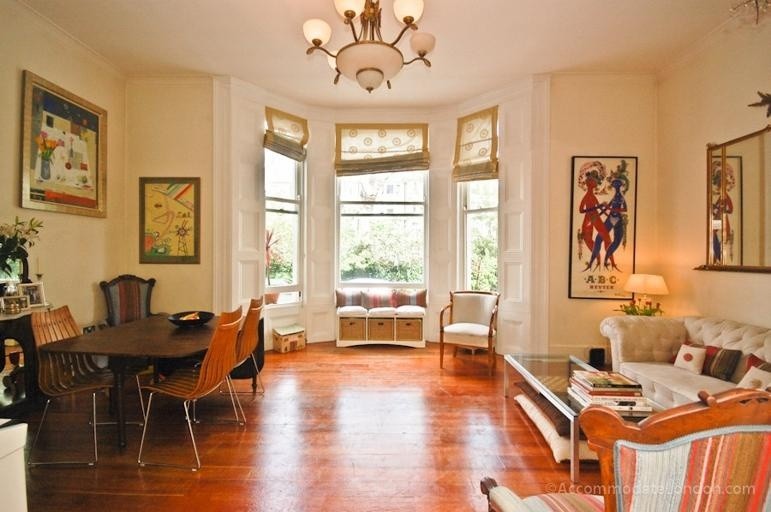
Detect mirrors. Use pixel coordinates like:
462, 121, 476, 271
692, 123, 771, 278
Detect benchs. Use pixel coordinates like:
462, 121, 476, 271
335, 304, 426, 351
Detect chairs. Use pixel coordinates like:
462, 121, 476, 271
134, 304, 244, 471
435, 289, 502, 376
477, 388, 771, 512
24, 305, 146, 468
98, 273, 162, 416
190, 293, 273, 428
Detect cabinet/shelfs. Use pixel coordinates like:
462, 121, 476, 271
0, 313, 39, 420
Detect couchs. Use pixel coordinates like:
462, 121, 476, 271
598, 311, 771, 410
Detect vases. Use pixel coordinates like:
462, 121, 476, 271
40, 156, 54, 181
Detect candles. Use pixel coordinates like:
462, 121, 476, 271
37, 257, 42, 274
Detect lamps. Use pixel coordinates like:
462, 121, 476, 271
298, 0, 439, 94
622, 271, 671, 305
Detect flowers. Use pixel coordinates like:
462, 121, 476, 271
0, 214, 48, 279
33, 129, 67, 167
615, 300, 664, 315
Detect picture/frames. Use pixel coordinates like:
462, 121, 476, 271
566, 152, 642, 303
16, 65, 109, 219
711, 154, 746, 268
16, 282, 46, 309
1, 294, 32, 311
135, 174, 205, 266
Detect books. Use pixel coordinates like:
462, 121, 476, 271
565, 370, 652, 419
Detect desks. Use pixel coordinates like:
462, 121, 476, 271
36, 315, 257, 451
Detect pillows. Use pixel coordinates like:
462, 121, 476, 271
332, 289, 361, 308
734, 365, 771, 393
390, 287, 428, 309
668, 339, 744, 382
672, 341, 710, 375
731, 354, 771, 385
359, 289, 394, 309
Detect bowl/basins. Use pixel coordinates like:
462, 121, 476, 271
168, 311, 216, 328
